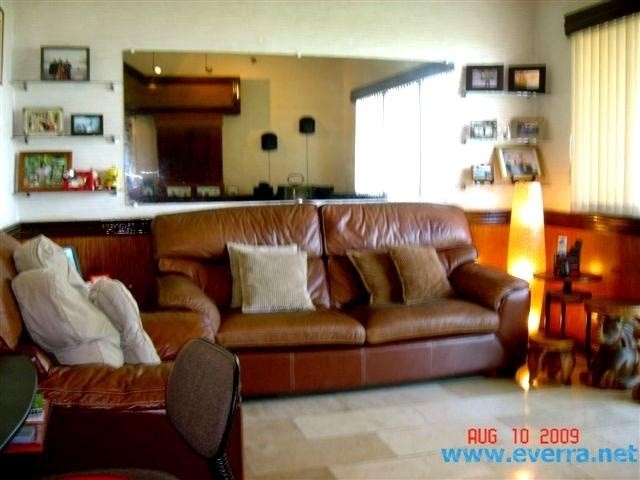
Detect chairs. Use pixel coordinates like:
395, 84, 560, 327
0, 232, 244, 480
42, 336, 241, 479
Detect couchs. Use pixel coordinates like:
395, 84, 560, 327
150, 203, 531, 397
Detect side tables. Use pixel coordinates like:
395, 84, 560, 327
579, 298, 640, 389
533, 272, 602, 371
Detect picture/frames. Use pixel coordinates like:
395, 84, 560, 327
0, 7, 103, 192
126, 173, 225, 202
467, 63, 546, 181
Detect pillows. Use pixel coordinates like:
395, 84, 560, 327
224, 242, 458, 314
11, 235, 162, 370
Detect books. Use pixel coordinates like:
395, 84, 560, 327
3, 393, 51, 453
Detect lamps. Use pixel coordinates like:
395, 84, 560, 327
260, 132, 277, 182
507, 181, 547, 380
299, 115, 315, 185
204, 54, 212, 73
152, 52, 162, 76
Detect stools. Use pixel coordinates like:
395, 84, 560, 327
528, 330, 577, 386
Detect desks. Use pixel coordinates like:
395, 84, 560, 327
0, 354, 49, 453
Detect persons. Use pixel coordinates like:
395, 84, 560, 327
36, 162, 46, 186
50, 57, 73, 80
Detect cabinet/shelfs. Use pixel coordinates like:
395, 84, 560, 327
10, 79, 124, 196
462, 88, 550, 188
